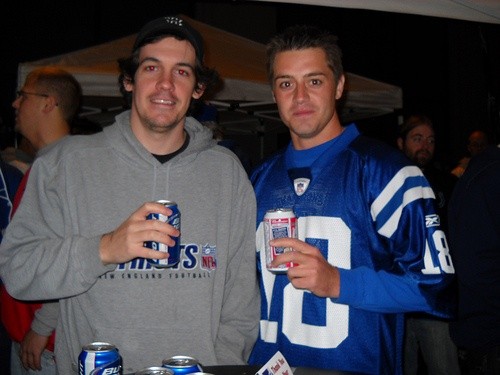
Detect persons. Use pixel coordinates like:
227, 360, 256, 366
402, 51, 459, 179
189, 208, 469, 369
0, 15, 260, 375
397, 113, 500, 375
249, 32, 457, 375
0, 67, 83, 375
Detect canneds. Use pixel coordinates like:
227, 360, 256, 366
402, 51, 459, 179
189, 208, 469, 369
263, 208, 300, 271
145, 200, 180, 267
134, 355, 214, 375
77, 342, 122, 375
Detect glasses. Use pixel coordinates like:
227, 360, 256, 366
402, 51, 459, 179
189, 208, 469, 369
18, 91, 59, 106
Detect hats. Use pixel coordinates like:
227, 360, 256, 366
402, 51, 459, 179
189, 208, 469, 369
131, 17, 204, 60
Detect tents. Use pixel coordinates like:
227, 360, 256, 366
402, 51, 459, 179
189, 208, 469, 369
15, 15, 403, 149
